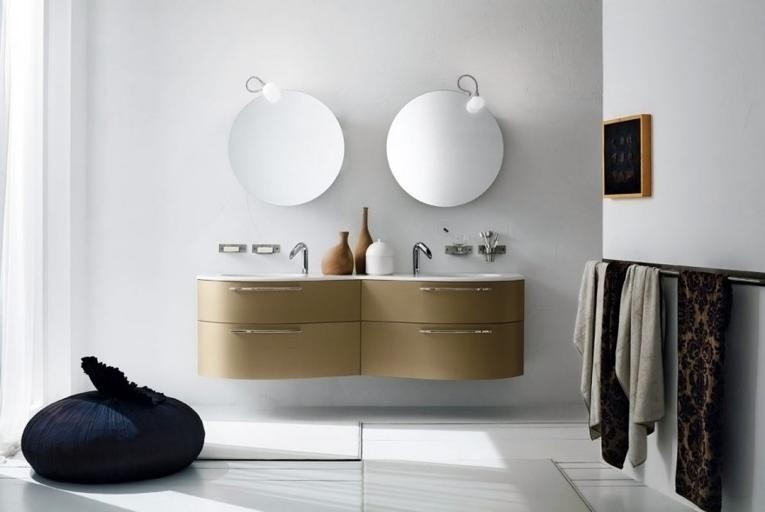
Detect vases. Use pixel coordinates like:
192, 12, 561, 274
320, 231, 353, 275
355, 207, 373, 276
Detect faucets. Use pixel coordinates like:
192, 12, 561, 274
411, 242, 433, 275
289, 243, 308, 273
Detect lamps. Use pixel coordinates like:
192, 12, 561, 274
457, 74, 484, 113
245, 76, 281, 102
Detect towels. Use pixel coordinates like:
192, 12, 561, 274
676, 268, 732, 512
573, 260, 609, 442
614, 264, 667, 468
600, 262, 629, 469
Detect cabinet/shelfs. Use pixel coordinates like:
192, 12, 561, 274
197, 279, 360, 381
360, 280, 525, 381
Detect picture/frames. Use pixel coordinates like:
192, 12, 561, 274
603, 113, 651, 200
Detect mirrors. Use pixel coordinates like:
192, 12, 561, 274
386, 90, 504, 207
228, 89, 345, 207
